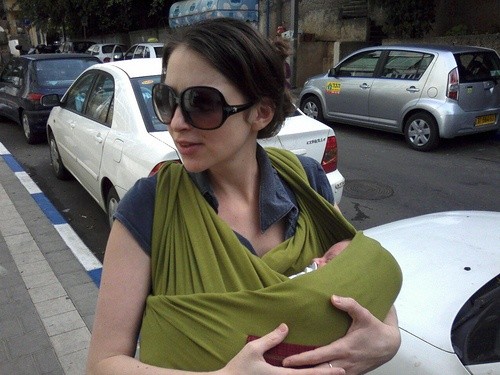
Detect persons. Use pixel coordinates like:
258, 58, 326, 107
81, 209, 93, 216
139, 229, 404, 375
85, 19, 401, 375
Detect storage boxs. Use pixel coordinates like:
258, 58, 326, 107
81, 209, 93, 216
168, 0, 258, 29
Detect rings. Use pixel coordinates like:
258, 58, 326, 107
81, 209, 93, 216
326, 360, 335, 369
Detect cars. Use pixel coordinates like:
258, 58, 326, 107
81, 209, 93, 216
356, 210, 500, 375
299, 44, 500, 151
26, 40, 165, 60
0, 53, 103, 145
46, 56, 345, 232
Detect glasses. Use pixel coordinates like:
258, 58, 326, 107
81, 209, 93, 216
151, 82, 264, 130
